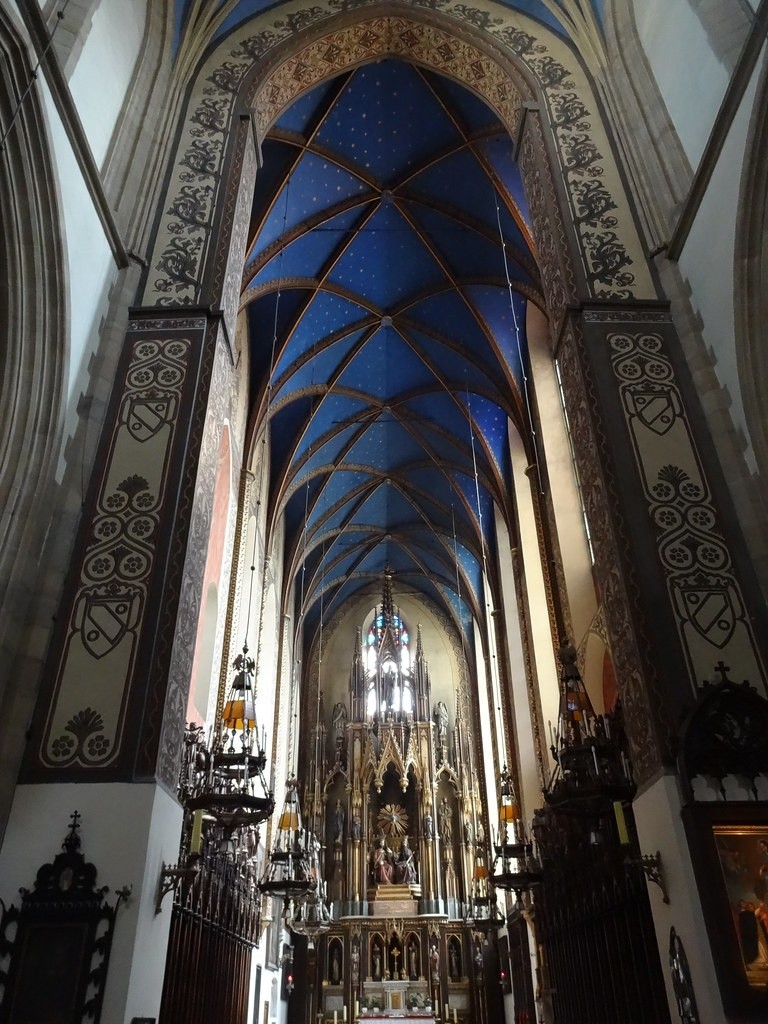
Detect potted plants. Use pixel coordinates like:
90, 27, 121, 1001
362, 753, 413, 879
423, 997, 433, 1011
411, 993, 422, 1012
369, 999, 381, 1013
358, 997, 369, 1013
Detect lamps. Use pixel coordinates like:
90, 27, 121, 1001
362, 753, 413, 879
489, 175, 636, 846
186, 144, 294, 854
449, 484, 506, 945
281, 943, 294, 1002
464, 359, 542, 910
497, 935, 512, 996
290, 528, 333, 951
257, 448, 318, 919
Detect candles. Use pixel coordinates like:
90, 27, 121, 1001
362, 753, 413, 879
356, 1001, 359, 1015
445, 1004, 449, 1020
435, 1000, 438, 1015
454, 1009, 457, 1023
334, 1010, 337, 1024
343, 1005, 347, 1021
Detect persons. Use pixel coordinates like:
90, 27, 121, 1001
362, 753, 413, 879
423, 810, 433, 840
476, 820, 485, 844
438, 795, 453, 845
464, 819, 474, 846
435, 701, 449, 745
331, 702, 347, 748
352, 812, 360, 842
394, 835, 418, 884
332, 798, 345, 845
330, 936, 483, 986
371, 839, 395, 885
384, 665, 396, 711
